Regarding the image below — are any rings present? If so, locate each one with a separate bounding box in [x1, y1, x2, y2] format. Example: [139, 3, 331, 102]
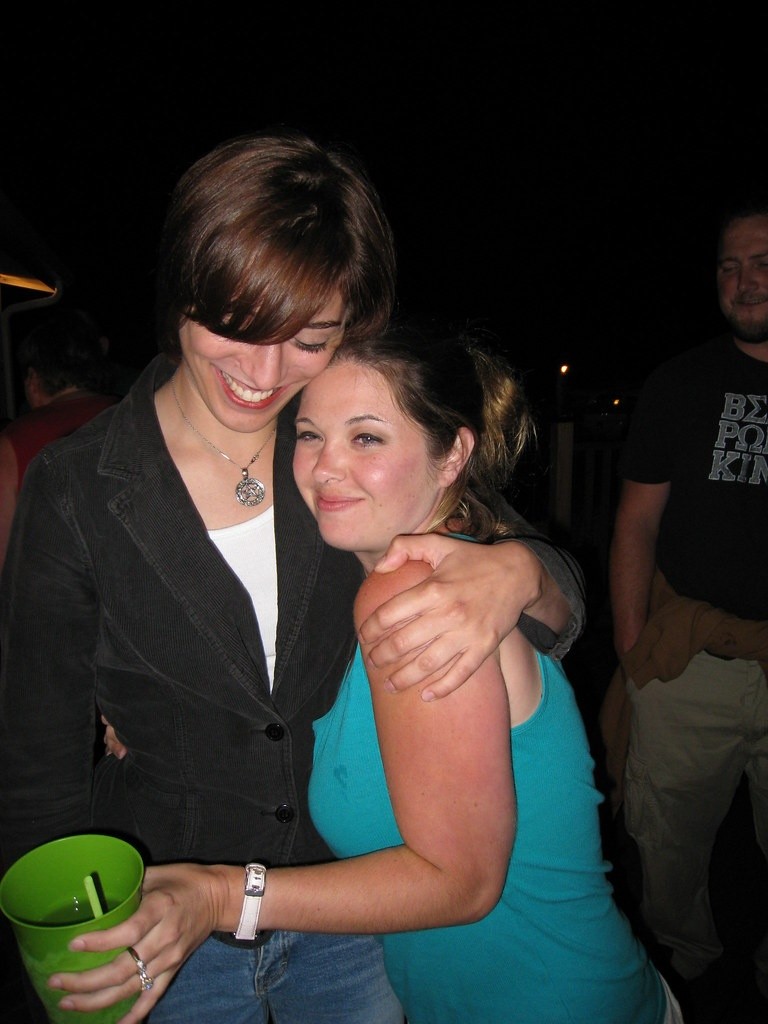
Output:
[126, 946, 145, 975]
[137, 969, 152, 990]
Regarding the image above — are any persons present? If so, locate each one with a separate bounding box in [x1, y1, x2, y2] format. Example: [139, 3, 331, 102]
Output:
[0, 310, 143, 592]
[604, 204, 768, 1024]
[0, 140, 583, 1024]
[48, 328, 683, 1024]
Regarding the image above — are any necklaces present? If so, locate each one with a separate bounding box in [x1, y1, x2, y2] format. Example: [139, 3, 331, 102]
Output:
[169, 375, 278, 507]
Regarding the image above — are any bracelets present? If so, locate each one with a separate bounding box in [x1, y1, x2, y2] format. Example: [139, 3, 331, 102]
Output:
[234, 862, 266, 941]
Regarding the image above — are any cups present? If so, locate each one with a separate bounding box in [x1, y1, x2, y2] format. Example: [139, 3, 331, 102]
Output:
[0, 836, 140, 1024]
